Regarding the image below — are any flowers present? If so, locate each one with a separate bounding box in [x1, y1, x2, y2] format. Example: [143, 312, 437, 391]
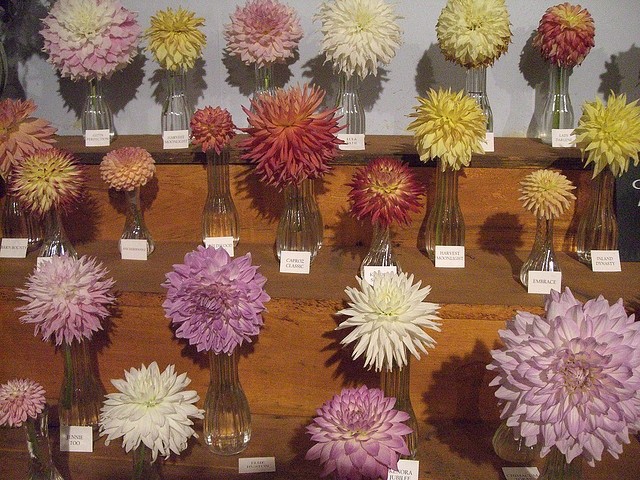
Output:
[14, 250, 124, 413]
[403, 85, 489, 245]
[333, 270, 443, 411]
[570, 88, 640, 253]
[487, 288, 640, 480]
[6, 148, 91, 256]
[435, 0, 513, 110]
[517, 170, 577, 269]
[0, 377, 46, 480]
[343, 154, 426, 252]
[38, 0, 143, 129]
[160, 245, 271, 435]
[189, 105, 236, 211]
[532, 2, 596, 129]
[304, 384, 414, 480]
[98, 361, 205, 480]
[0, 98, 59, 237]
[233, 81, 350, 249]
[312, 0, 405, 121]
[222, 0, 304, 91]
[100, 146, 156, 239]
[143, 5, 207, 116]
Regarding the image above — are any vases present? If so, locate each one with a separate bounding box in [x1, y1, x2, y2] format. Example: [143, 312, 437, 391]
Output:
[577, 169, 619, 264]
[426, 157, 465, 260]
[275, 179, 323, 266]
[330, 73, 365, 150]
[533, 446, 583, 480]
[541, 67, 574, 145]
[203, 349, 252, 455]
[57, 338, 108, 431]
[131, 443, 160, 480]
[118, 187, 154, 257]
[492, 417, 545, 464]
[2, 183, 45, 252]
[360, 222, 402, 278]
[81, 81, 115, 143]
[161, 72, 194, 145]
[462, 67, 493, 132]
[520, 216, 561, 288]
[37, 207, 78, 261]
[380, 350, 419, 459]
[249, 62, 275, 128]
[203, 149, 240, 247]
[22, 406, 65, 480]
